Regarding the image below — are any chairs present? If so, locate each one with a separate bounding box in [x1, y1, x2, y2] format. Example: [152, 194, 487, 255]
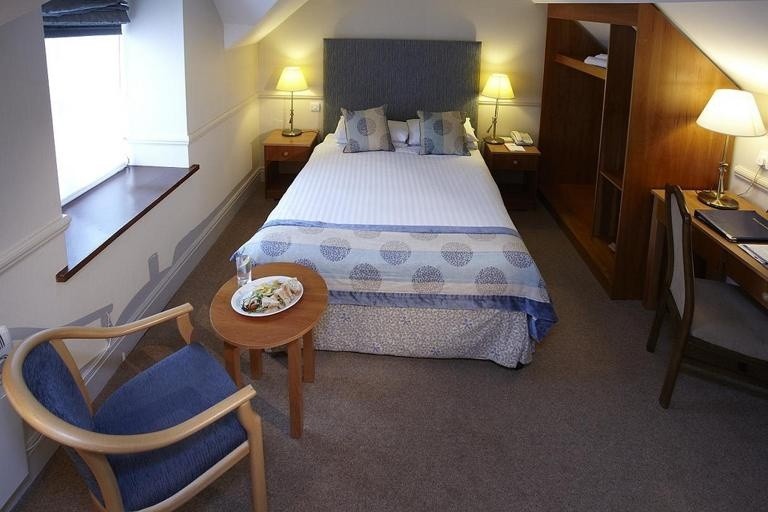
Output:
[2, 302, 268, 511]
[646, 183, 767, 410]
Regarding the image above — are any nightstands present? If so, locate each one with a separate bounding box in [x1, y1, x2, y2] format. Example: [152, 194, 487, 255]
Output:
[482, 137, 541, 212]
[261, 129, 317, 202]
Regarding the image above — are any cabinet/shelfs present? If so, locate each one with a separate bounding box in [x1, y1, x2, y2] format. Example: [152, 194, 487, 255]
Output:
[536, 5, 741, 302]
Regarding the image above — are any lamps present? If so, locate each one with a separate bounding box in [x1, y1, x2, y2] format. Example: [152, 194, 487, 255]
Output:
[481, 73, 516, 145]
[274, 66, 309, 137]
[694, 88, 767, 211]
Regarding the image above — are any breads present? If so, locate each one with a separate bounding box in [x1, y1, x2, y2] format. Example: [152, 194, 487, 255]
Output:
[257, 277, 300, 313]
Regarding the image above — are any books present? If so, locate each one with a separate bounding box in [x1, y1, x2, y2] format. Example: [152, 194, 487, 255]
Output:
[503, 142, 526, 153]
[736, 242, 768, 269]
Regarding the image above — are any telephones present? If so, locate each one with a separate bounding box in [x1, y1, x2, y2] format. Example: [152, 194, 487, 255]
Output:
[511, 131, 533, 146]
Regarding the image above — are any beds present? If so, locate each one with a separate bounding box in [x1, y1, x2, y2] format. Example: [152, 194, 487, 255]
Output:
[235, 118, 541, 372]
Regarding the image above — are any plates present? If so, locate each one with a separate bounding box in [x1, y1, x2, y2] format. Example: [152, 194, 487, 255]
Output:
[230, 275, 304, 318]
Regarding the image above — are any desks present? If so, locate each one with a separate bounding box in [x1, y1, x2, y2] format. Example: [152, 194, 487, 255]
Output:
[208, 262, 331, 441]
[641, 188, 767, 311]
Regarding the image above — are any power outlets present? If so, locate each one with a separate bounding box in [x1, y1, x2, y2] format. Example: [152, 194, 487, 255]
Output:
[754, 149, 767, 171]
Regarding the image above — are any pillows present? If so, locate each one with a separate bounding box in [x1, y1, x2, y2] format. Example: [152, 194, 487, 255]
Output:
[332, 103, 479, 157]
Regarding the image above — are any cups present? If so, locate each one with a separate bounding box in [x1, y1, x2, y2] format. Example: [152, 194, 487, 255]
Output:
[235, 254, 252, 288]
